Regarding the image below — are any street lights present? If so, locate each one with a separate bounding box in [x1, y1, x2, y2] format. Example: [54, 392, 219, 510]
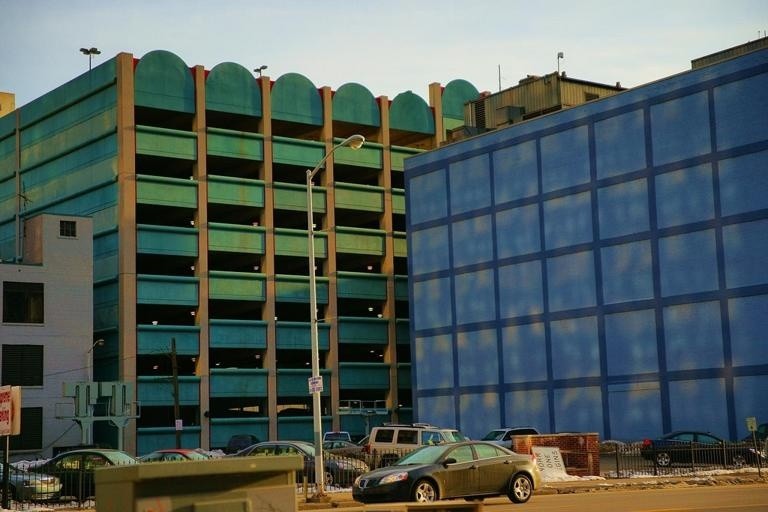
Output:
[306, 131, 367, 500]
[87, 337, 106, 444]
[79, 47, 101, 70]
[253, 65, 268, 77]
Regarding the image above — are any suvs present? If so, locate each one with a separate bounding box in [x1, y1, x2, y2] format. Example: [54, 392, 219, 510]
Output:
[364, 423, 468, 469]
[477, 425, 542, 448]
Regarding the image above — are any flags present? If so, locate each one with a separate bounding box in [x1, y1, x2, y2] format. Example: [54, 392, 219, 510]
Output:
[558, 53, 563, 58]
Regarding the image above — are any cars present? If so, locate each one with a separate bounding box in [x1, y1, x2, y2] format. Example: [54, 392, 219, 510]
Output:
[640, 428, 766, 469]
[27, 447, 141, 502]
[230, 438, 367, 488]
[321, 430, 353, 444]
[134, 447, 211, 462]
[0, 459, 65, 505]
[339, 398, 387, 410]
[351, 439, 542, 506]
